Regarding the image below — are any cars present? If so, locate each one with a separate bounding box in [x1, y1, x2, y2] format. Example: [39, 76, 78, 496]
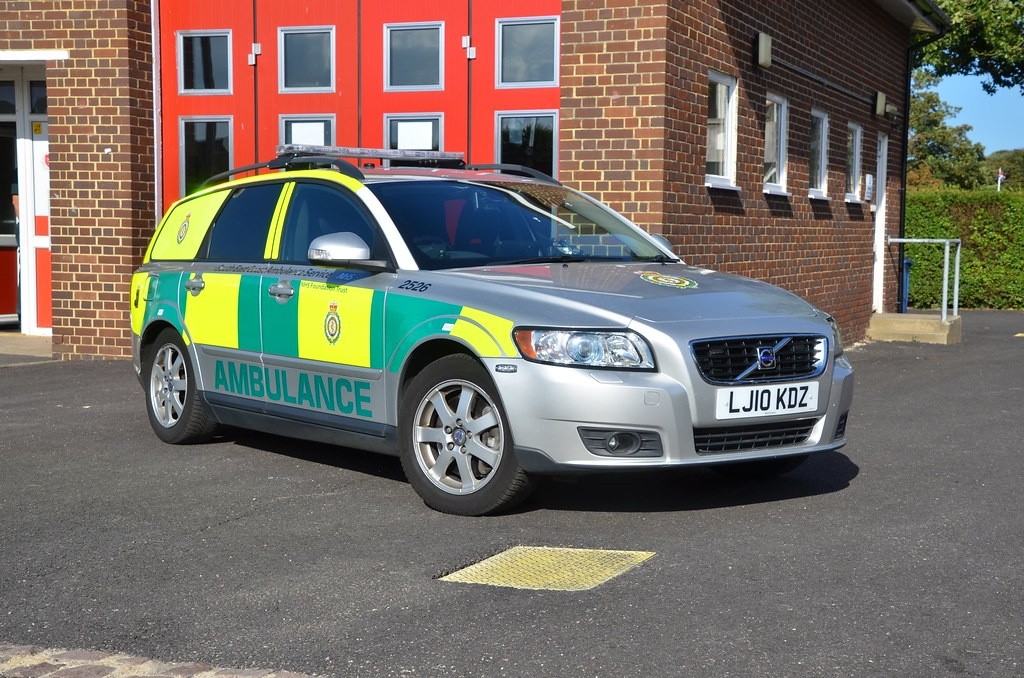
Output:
[129, 144, 856, 517]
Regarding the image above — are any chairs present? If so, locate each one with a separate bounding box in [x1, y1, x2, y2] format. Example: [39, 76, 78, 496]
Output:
[304, 195, 504, 267]
